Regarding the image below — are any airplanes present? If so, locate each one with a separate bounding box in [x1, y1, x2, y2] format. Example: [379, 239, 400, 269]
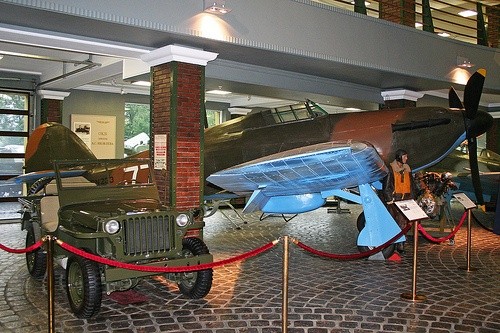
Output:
[22, 69, 494, 217]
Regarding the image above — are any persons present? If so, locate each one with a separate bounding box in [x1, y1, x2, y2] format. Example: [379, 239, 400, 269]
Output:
[385, 150, 416, 255]
[76, 125, 90, 132]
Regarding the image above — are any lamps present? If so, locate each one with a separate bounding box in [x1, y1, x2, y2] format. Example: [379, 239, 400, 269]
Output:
[456, 56, 476, 68]
[203, 1, 233, 15]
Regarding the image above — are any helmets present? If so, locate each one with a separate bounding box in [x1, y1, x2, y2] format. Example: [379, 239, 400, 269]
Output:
[395, 149, 407, 164]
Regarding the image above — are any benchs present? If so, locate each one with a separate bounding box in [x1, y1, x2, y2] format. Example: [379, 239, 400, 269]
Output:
[39, 176, 96, 231]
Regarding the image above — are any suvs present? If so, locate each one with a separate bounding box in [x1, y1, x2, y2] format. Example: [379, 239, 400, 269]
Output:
[17, 158, 213, 320]
